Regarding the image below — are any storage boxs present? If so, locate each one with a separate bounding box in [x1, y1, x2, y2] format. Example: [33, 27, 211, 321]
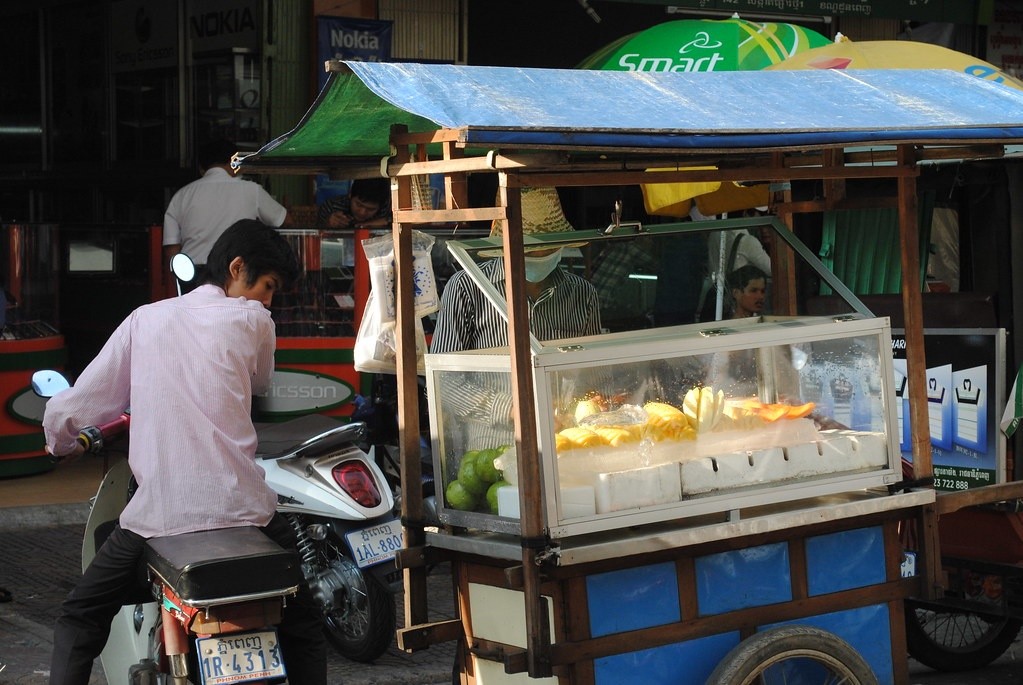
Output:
[595, 461, 680, 514]
[498, 485, 596, 522]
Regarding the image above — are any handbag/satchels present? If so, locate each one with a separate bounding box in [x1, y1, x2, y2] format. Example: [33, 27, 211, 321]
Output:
[354, 287, 428, 376]
[698, 232, 745, 323]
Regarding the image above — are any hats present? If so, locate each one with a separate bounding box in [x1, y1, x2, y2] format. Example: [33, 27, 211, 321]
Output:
[477, 185, 589, 257]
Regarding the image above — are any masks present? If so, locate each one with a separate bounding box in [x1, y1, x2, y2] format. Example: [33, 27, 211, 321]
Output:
[524, 246, 563, 283]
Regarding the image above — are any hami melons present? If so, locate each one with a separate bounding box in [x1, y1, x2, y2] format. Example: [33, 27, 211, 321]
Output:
[743, 399, 815, 423]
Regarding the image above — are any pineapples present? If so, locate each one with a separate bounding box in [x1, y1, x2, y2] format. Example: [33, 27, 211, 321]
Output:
[554, 397, 698, 452]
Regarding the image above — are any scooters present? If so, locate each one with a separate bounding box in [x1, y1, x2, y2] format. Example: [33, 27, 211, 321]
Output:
[253, 414, 409, 662]
[32, 254, 304, 685]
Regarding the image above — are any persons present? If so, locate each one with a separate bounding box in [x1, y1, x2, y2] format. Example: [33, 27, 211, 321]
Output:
[757, 225, 822, 316]
[316, 178, 395, 294]
[162, 141, 294, 297]
[694, 209, 772, 323]
[584, 236, 657, 321]
[423, 184, 617, 451]
[726, 266, 767, 319]
[41, 218, 330, 685]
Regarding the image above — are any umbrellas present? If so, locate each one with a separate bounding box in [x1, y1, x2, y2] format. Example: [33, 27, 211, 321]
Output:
[641, 31, 1023, 217]
[575, 20, 835, 71]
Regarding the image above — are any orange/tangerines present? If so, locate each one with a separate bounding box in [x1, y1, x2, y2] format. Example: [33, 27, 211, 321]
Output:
[446, 444, 509, 515]
